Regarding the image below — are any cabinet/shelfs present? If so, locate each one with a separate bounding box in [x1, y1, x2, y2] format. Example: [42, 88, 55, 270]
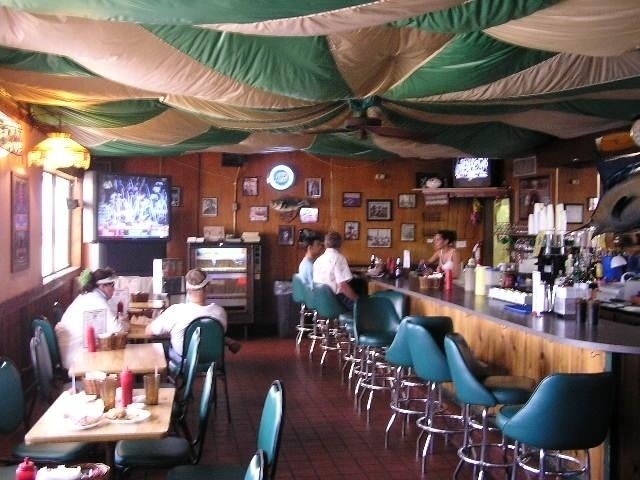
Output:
[368, 276, 640, 480]
[89, 242, 166, 276]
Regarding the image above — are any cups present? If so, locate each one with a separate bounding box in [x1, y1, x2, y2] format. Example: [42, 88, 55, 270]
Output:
[144, 373, 160, 404]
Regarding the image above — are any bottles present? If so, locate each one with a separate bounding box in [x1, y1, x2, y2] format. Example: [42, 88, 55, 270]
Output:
[464, 258, 475, 292]
[370, 254, 375, 269]
[395, 258, 401, 278]
[121, 366, 133, 406]
[15, 457, 35, 480]
[87, 321, 95, 352]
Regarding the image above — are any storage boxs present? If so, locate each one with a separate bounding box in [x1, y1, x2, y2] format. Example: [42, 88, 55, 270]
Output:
[513, 156, 544, 176]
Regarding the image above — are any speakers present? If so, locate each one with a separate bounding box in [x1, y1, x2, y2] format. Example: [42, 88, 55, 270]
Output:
[489, 156, 505, 187]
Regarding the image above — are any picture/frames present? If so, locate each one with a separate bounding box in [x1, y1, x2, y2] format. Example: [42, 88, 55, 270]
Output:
[10, 170, 30, 273]
[400, 223, 416, 242]
[304, 177, 321, 198]
[367, 199, 393, 221]
[344, 221, 360, 240]
[242, 176, 259, 196]
[564, 203, 584, 224]
[367, 228, 392, 248]
[299, 208, 318, 223]
[201, 198, 217, 217]
[398, 193, 417, 209]
[278, 225, 295, 245]
[518, 174, 551, 220]
[171, 187, 180, 207]
[248, 205, 269, 222]
[343, 192, 361, 207]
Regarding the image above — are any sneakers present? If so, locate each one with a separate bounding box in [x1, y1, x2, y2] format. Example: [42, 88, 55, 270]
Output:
[229, 342, 241, 354]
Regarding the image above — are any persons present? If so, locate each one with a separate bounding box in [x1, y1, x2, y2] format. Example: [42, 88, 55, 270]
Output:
[419, 229, 464, 280]
[146, 266, 242, 375]
[54, 266, 131, 383]
[313, 232, 360, 312]
[299, 236, 323, 291]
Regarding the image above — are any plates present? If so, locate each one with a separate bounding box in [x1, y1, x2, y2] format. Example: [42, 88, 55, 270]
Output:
[104, 409, 151, 423]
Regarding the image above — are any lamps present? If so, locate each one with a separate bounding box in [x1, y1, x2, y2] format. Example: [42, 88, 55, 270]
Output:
[266, 162, 296, 192]
[27, 113, 90, 169]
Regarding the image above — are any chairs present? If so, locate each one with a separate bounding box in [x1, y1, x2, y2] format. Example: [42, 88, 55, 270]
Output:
[172, 327, 201, 460]
[312, 282, 349, 369]
[168, 380, 285, 480]
[339, 312, 372, 390]
[292, 273, 314, 352]
[244, 450, 267, 480]
[30, 326, 71, 416]
[168, 317, 232, 425]
[303, 285, 325, 360]
[385, 316, 453, 449]
[115, 362, 216, 472]
[405, 321, 489, 478]
[496, 371, 615, 480]
[30, 316, 77, 389]
[354, 297, 411, 425]
[346, 290, 405, 409]
[0, 359, 99, 466]
[54, 302, 62, 322]
[445, 333, 536, 480]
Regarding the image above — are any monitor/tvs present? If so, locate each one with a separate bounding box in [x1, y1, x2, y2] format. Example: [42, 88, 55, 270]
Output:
[96, 171, 172, 244]
[451, 154, 492, 188]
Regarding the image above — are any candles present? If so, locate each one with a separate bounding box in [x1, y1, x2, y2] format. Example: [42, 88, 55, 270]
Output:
[446, 269, 453, 290]
[87, 324, 97, 352]
[119, 364, 133, 407]
[16, 458, 36, 479]
[117, 300, 123, 318]
